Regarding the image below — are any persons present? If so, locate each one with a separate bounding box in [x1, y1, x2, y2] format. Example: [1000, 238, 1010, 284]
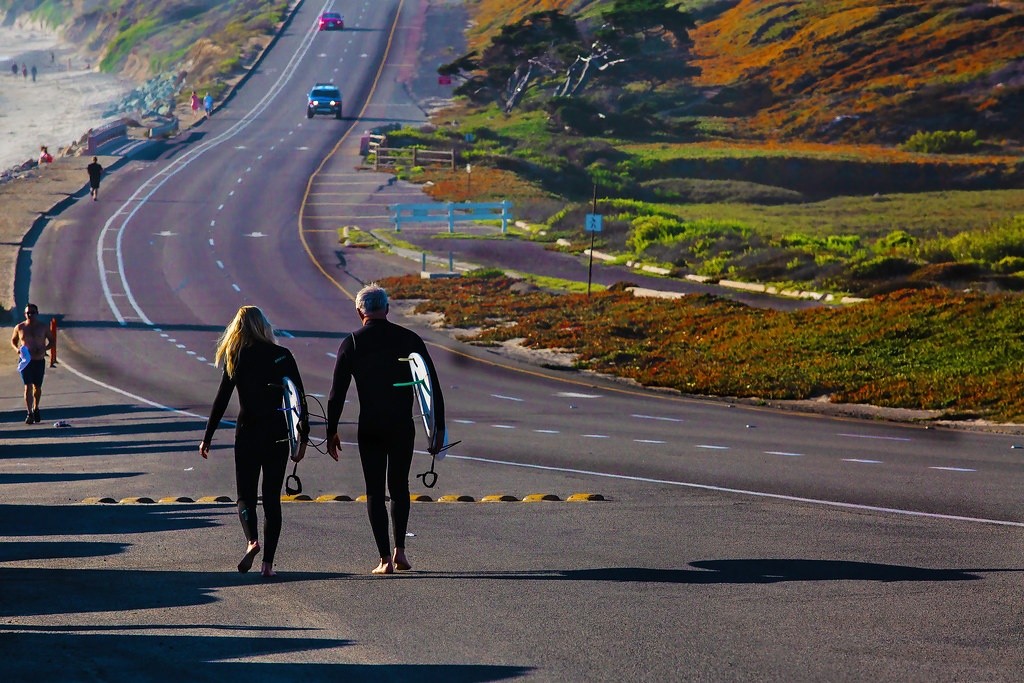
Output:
[325, 284, 447, 575]
[190, 91, 200, 119]
[11, 52, 91, 87]
[11, 302, 56, 425]
[87, 156, 104, 201]
[199, 306, 310, 579]
[37, 145, 53, 166]
[203, 91, 213, 120]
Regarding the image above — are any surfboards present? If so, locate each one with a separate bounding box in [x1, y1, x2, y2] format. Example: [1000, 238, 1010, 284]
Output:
[267, 377, 302, 459]
[392, 352, 450, 462]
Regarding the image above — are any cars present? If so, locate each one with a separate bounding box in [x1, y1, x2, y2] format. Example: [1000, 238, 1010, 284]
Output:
[317, 12, 344, 31]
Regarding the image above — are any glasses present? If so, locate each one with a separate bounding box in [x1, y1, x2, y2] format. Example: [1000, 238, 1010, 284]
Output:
[27, 312, 35, 314]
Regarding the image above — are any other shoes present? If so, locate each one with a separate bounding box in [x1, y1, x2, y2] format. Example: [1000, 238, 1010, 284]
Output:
[34, 410, 40, 422]
[25, 413, 34, 424]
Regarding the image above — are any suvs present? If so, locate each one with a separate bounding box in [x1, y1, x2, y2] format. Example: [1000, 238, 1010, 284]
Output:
[306, 84, 345, 118]
